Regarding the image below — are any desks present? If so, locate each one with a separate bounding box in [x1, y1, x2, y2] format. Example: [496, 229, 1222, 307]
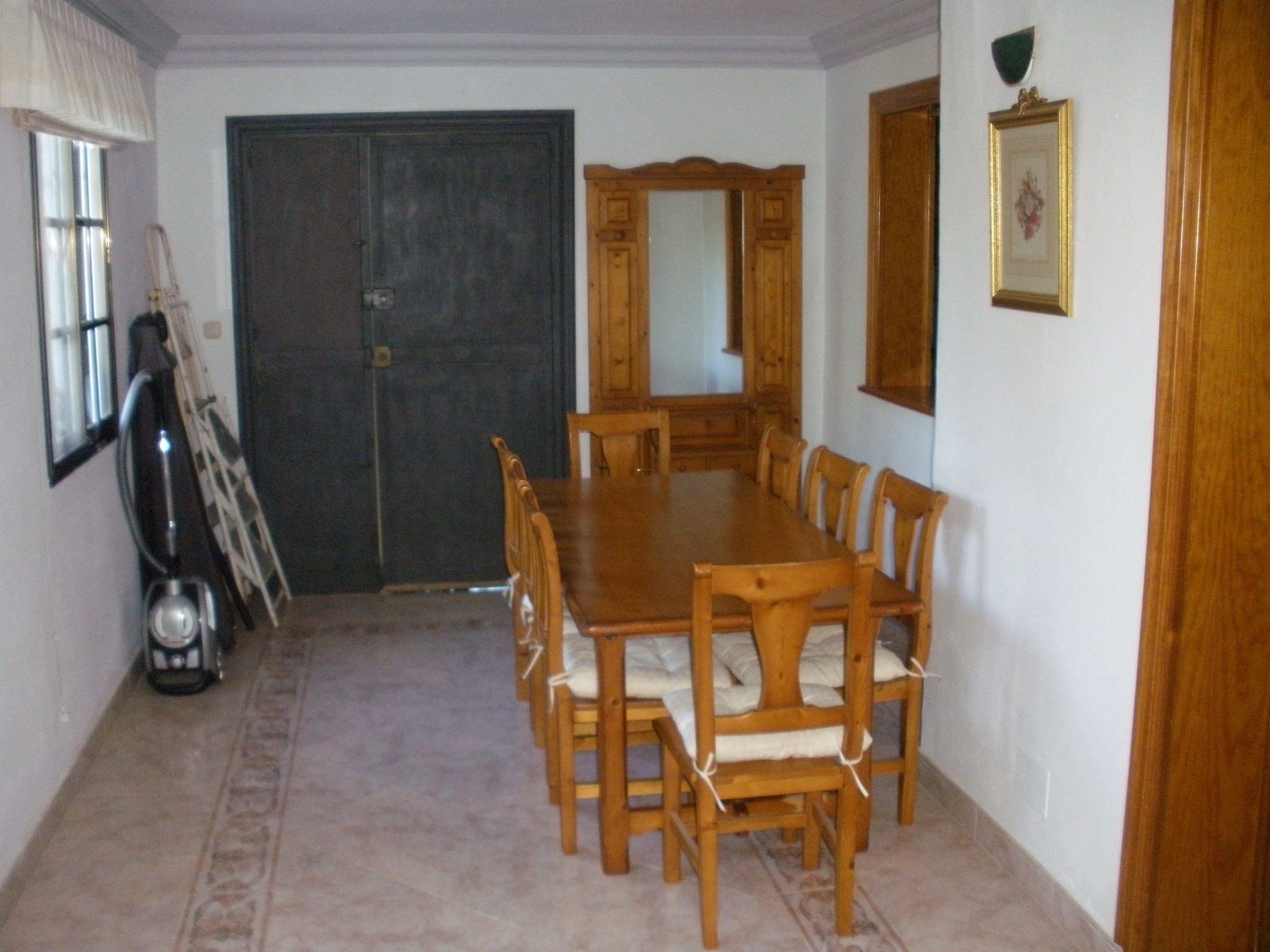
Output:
[522, 468, 927, 875]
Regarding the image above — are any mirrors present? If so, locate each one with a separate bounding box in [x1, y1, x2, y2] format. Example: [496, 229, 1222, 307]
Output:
[647, 187, 746, 399]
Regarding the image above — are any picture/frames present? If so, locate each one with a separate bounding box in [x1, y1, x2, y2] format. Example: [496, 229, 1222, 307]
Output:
[988, 85, 1074, 318]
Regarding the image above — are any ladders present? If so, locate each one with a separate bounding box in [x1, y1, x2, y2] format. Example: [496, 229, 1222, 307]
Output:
[144, 222, 292, 627]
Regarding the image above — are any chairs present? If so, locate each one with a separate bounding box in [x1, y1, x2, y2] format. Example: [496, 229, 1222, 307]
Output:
[489, 408, 949, 952]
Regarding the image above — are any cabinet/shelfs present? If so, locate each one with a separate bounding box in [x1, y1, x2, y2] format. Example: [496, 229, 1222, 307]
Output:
[587, 157, 806, 481]
